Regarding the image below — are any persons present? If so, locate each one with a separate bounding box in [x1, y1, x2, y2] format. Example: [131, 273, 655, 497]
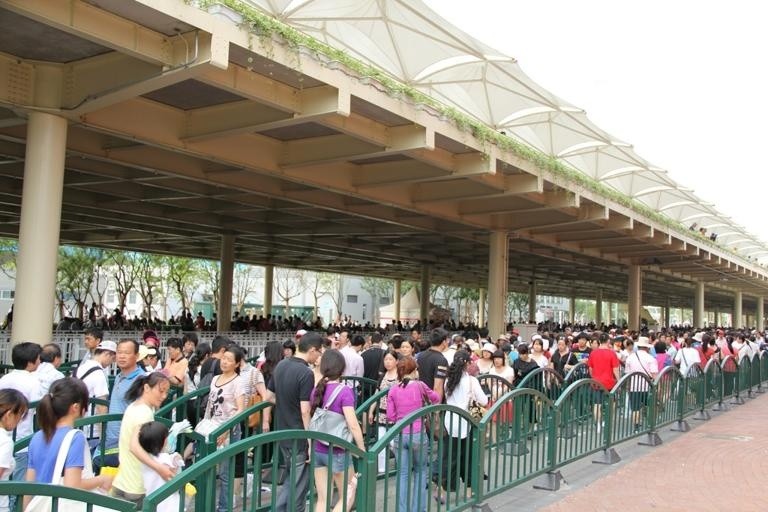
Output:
[689, 222, 763, 268]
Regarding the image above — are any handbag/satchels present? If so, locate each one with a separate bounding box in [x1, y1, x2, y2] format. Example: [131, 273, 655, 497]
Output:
[466, 371, 488, 424]
[193, 418, 226, 451]
[197, 358, 220, 412]
[306, 383, 354, 449]
[563, 363, 580, 380]
[415, 380, 450, 440]
[21, 428, 122, 512]
[245, 392, 261, 427]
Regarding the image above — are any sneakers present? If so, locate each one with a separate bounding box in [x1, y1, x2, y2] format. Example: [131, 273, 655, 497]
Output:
[465, 496, 475, 502]
[633, 424, 640, 433]
[596, 421, 605, 434]
[433, 490, 445, 505]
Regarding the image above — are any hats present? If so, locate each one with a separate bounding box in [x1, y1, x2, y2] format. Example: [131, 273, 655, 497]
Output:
[636, 336, 654, 349]
[96, 340, 118, 353]
[496, 327, 521, 343]
[691, 332, 703, 343]
[136, 344, 157, 362]
[480, 342, 498, 354]
[295, 328, 308, 340]
[529, 334, 550, 350]
